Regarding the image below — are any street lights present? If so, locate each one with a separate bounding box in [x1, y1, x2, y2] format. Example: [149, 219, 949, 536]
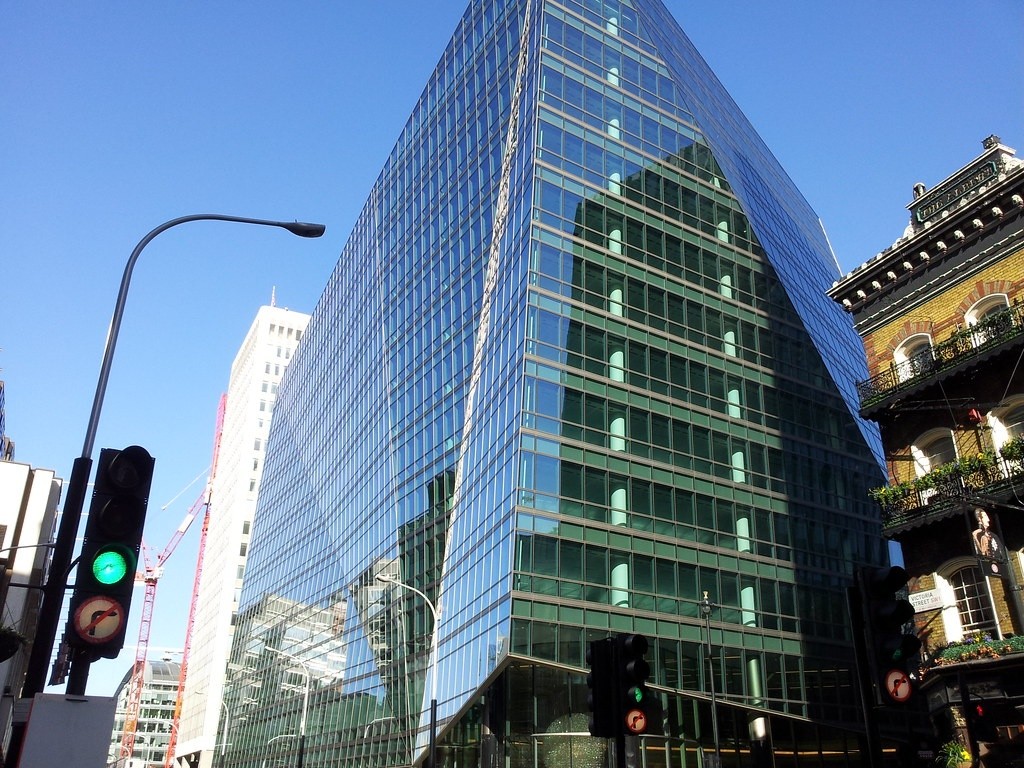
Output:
[110, 718, 180, 768]
[195, 689, 230, 768]
[265, 646, 309, 765]
[375, 572, 439, 768]
[0, 212, 326, 768]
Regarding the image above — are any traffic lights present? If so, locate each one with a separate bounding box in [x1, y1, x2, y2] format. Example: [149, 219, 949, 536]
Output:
[868, 564, 924, 683]
[611, 633, 650, 739]
[70, 446, 159, 664]
